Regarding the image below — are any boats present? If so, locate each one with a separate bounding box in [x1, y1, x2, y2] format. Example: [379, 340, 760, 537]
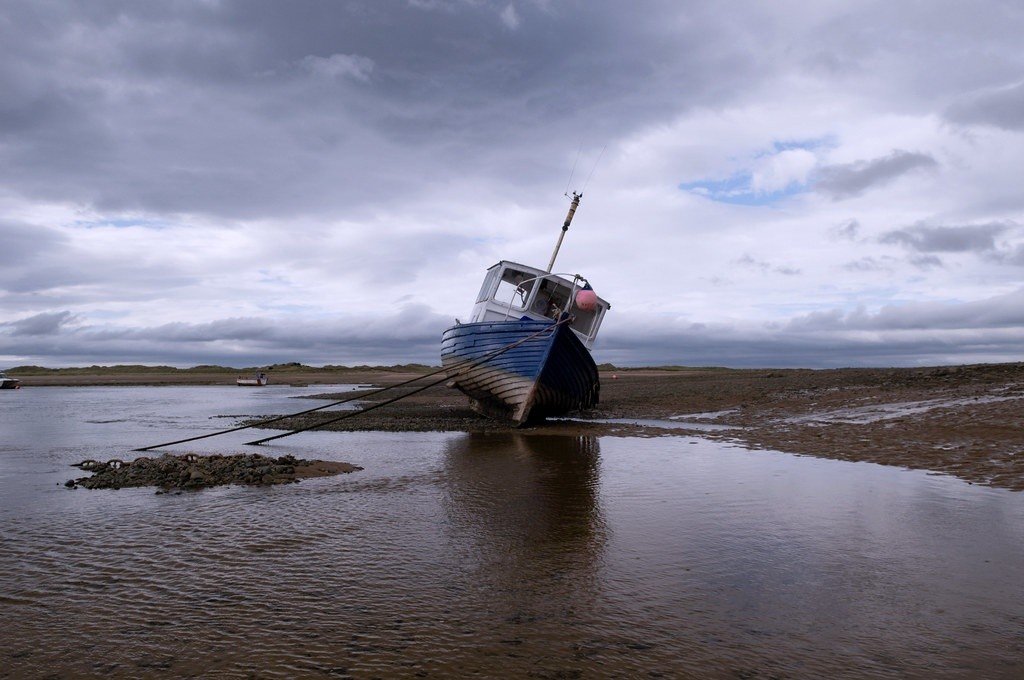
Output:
[0, 373, 21, 390]
[440, 179, 616, 430]
[236, 376, 268, 386]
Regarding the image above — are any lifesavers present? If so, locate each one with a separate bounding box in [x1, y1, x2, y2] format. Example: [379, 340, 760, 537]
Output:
[534, 298, 546, 311]
